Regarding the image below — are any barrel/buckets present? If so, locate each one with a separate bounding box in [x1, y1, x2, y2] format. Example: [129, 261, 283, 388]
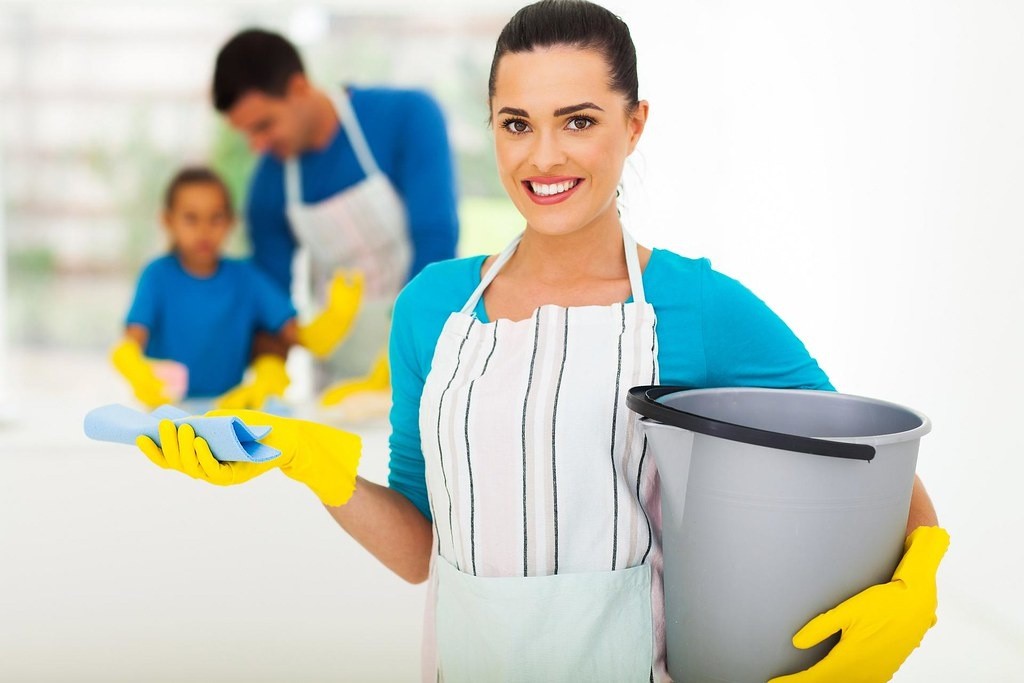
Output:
[625, 384, 933, 683]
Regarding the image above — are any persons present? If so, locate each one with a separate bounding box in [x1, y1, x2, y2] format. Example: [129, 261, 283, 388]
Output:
[136, 1, 950, 683]
[109, 170, 362, 410]
[209, 28, 460, 410]
